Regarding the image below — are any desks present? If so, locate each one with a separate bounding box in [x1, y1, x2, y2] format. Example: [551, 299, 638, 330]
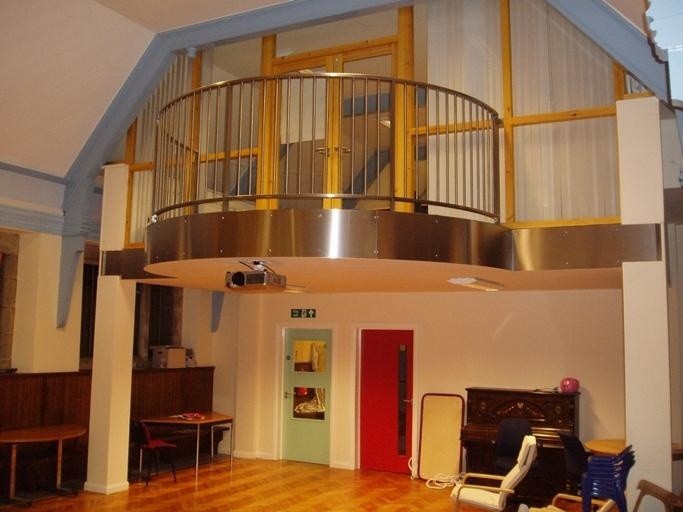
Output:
[582, 439, 683, 463]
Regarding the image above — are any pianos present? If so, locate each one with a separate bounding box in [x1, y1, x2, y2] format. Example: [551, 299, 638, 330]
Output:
[459, 387, 582, 507]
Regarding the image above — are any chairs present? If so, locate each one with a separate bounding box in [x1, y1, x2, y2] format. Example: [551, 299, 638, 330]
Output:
[491, 416, 531, 505]
[130, 420, 178, 487]
[450, 435, 538, 511]
[581, 445, 635, 512]
[557, 429, 591, 493]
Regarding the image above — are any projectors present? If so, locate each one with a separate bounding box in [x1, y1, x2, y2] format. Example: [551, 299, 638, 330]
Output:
[224, 270, 285, 293]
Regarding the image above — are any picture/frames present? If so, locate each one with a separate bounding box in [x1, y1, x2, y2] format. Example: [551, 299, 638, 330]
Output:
[292, 340, 326, 372]
[291, 386, 326, 422]
